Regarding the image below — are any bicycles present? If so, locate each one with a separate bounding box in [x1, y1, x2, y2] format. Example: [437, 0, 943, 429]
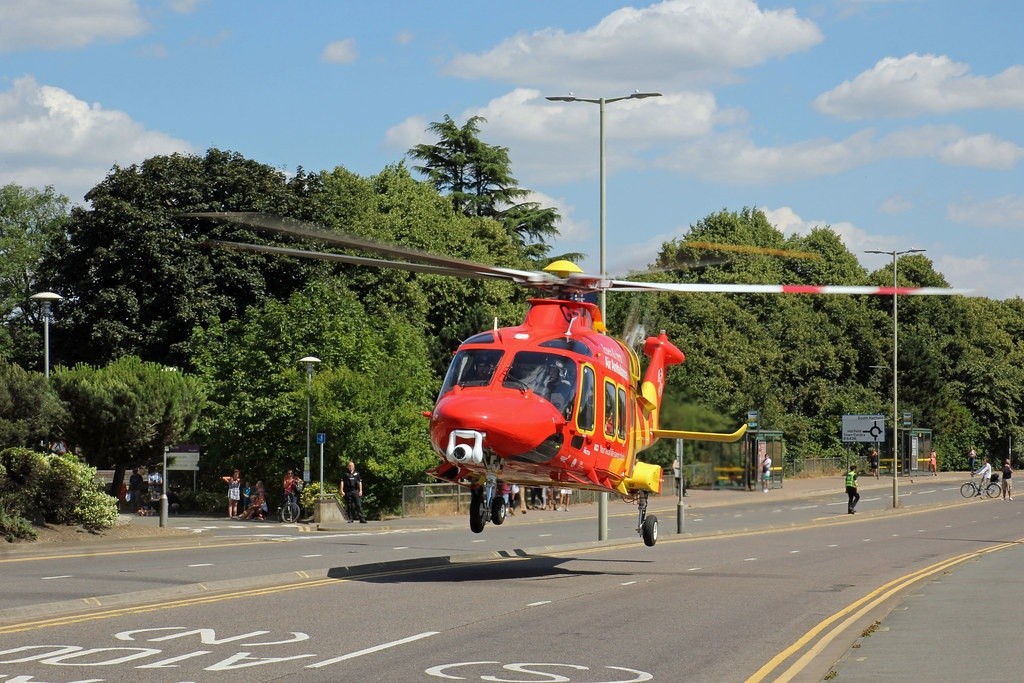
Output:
[960, 472, 1002, 498]
[280, 488, 301, 523]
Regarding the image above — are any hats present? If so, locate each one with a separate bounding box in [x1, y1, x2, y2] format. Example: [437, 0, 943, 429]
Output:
[850, 466, 856, 469]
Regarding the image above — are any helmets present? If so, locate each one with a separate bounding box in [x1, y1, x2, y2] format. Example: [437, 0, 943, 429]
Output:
[474, 355, 493, 375]
[548, 360, 567, 379]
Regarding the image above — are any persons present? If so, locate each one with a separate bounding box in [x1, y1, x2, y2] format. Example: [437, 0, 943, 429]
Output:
[632, 489, 638, 504]
[118, 483, 127, 510]
[237, 481, 268, 520]
[222, 469, 241, 519]
[340, 462, 367, 523]
[1001, 459, 1014, 500]
[929, 448, 937, 476]
[496, 483, 572, 515]
[845, 466, 860, 514]
[869, 448, 877, 477]
[968, 448, 975, 472]
[128, 469, 144, 513]
[974, 458, 991, 499]
[141, 488, 181, 515]
[284, 469, 303, 518]
[762, 454, 771, 493]
[673, 456, 687, 497]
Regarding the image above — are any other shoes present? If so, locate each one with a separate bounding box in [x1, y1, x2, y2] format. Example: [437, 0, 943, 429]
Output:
[849, 507, 856, 512]
[974, 493, 981, 497]
[935, 473, 937, 476]
[226, 513, 265, 522]
[683, 492, 689, 497]
[763, 488, 768, 493]
[505, 505, 570, 514]
[1009, 497, 1013, 501]
[348, 518, 353, 523]
[1001, 498, 1005, 500]
[360, 518, 367, 523]
[848, 509, 854, 514]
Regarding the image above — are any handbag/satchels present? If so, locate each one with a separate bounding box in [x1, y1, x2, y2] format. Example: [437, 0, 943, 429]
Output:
[990, 474, 999, 482]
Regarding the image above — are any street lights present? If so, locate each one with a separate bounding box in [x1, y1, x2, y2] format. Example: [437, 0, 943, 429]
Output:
[299, 356, 322, 519]
[863, 250, 929, 508]
[29, 291, 64, 382]
[544, 92, 666, 528]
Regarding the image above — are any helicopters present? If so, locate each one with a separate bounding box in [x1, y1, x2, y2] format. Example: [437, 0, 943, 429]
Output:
[177, 209, 1024, 547]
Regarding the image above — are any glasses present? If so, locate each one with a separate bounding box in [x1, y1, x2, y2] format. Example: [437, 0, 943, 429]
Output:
[287, 472, 293, 474]
